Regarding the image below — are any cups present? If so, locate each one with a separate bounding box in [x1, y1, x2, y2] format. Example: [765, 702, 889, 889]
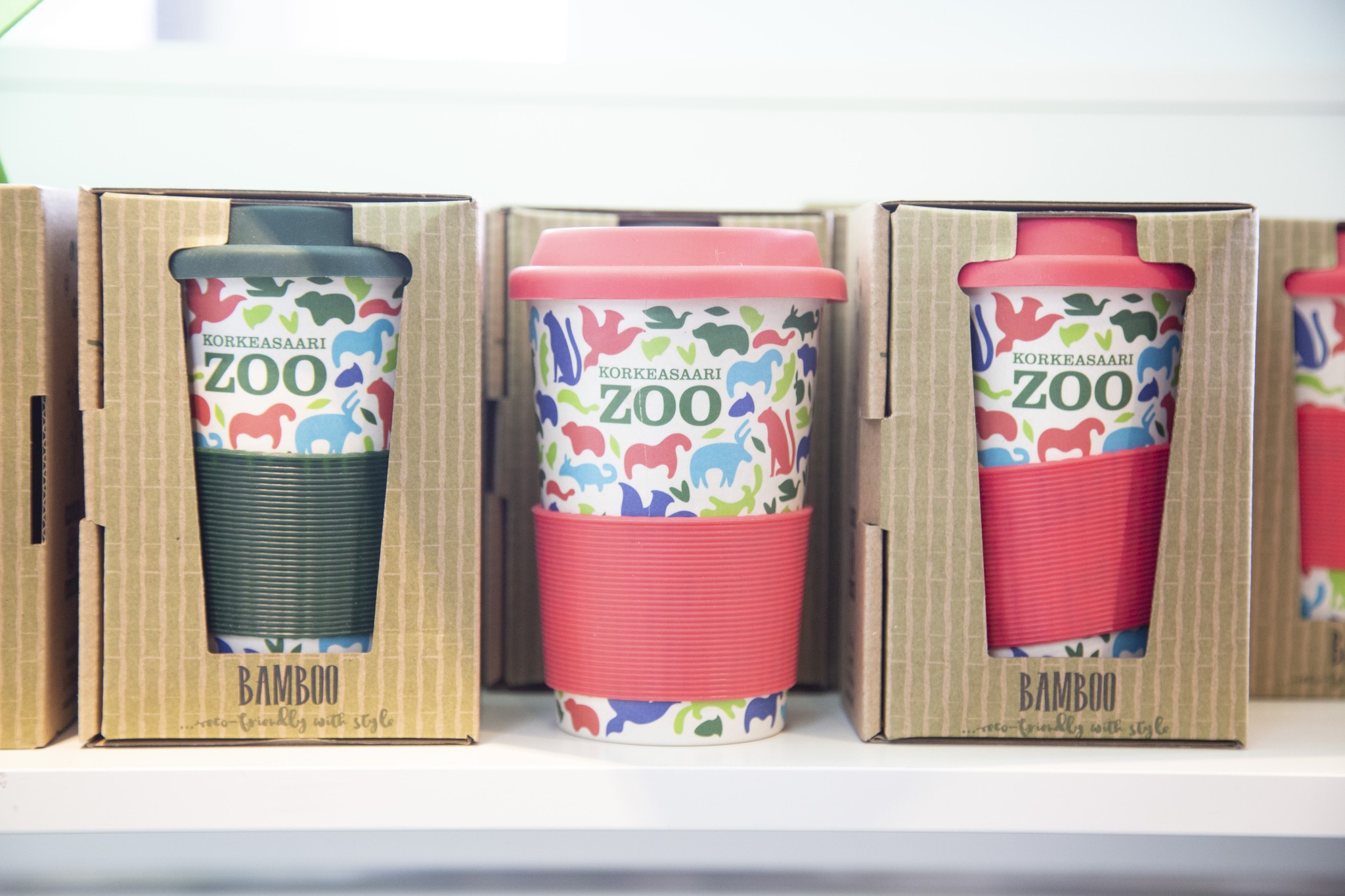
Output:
[504, 224, 848, 749]
[1284, 227, 1345, 628]
[168, 202, 412, 656]
[948, 216, 1195, 661]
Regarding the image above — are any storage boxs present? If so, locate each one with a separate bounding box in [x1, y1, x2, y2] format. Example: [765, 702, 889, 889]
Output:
[0, 181, 1345, 751]
